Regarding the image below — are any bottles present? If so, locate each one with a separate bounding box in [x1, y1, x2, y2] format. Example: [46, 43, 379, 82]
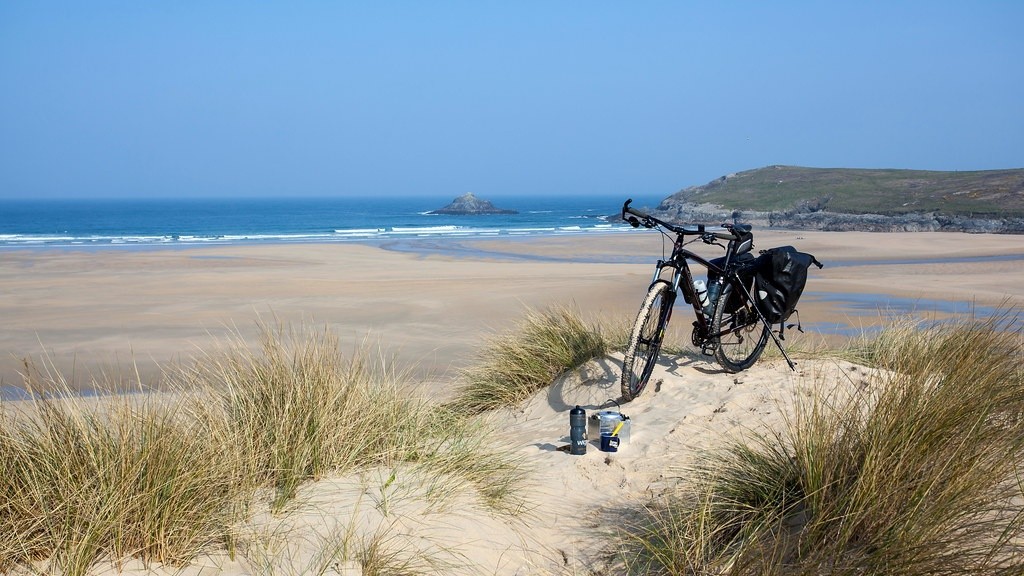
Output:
[569, 405, 587, 455]
[693, 280, 710, 307]
[704, 278, 721, 321]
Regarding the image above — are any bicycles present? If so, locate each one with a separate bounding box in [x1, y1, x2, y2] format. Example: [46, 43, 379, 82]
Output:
[621, 198, 774, 404]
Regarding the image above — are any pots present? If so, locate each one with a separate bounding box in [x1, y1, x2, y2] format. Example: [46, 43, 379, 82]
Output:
[588, 410, 625, 442]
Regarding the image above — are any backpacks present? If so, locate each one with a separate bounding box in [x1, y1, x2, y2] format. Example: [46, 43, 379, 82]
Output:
[754, 246, 823, 340]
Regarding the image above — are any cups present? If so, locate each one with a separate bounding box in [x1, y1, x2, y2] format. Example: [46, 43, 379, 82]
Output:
[618, 419, 630, 445]
[600, 431, 620, 452]
